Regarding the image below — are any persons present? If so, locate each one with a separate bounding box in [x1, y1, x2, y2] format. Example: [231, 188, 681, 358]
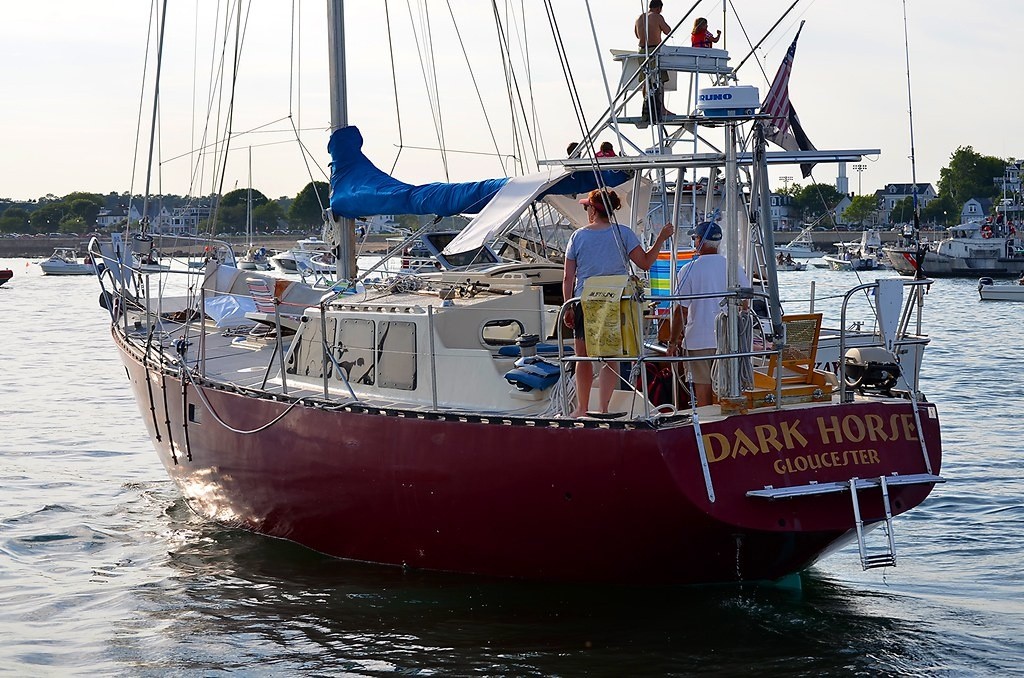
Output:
[986, 214, 994, 223]
[595, 141, 622, 158]
[249, 244, 256, 256]
[567, 143, 582, 157]
[785, 253, 791, 265]
[953, 230, 967, 238]
[996, 213, 1005, 225]
[857, 248, 861, 259]
[692, 17, 721, 49]
[634, 0, 676, 116]
[667, 221, 751, 407]
[561, 189, 673, 419]
[260, 245, 266, 256]
[777, 252, 783, 265]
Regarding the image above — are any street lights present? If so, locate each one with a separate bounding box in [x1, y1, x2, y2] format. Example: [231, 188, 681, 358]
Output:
[778, 176, 794, 193]
[852, 164, 868, 196]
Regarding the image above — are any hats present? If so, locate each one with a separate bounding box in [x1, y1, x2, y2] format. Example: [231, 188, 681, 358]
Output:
[687, 222, 722, 241]
[579, 196, 607, 214]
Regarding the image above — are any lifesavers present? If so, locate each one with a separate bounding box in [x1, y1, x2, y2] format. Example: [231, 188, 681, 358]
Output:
[981, 226, 991, 239]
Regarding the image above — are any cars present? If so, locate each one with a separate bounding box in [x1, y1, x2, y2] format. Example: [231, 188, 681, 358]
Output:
[873, 224, 882, 230]
[837, 224, 849, 231]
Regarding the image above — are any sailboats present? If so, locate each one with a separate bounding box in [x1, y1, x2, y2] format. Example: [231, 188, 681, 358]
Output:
[86, 0, 939, 585]
[236, 146, 270, 270]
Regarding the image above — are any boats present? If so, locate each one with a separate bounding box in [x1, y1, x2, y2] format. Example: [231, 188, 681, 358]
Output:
[882, 165, 1024, 278]
[977, 277, 1024, 302]
[189, 258, 237, 269]
[776, 261, 809, 272]
[128, 248, 171, 273]
[774, 224, 826, 258]
[1, 269, 14, 285]
[272, 237, 338, 274]
[384, 236, 443, 276]
[39, 249, 103, 275]
[824, 242, 878, 271]
[310, 253, 339, 275]
[811, 263, 829, 268]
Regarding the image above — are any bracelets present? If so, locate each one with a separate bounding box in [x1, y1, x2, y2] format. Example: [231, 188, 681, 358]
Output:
[562, 305, 572, 310]
[667, 340, 677, 347]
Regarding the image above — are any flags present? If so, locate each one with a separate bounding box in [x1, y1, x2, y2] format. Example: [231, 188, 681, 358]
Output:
[752, 35, 818, 178]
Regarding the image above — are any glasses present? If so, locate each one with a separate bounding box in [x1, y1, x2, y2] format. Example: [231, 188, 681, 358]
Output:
[582, 204, 589, 211]
[691, 236, 698, 240]
[704, 23, 708, 27]
